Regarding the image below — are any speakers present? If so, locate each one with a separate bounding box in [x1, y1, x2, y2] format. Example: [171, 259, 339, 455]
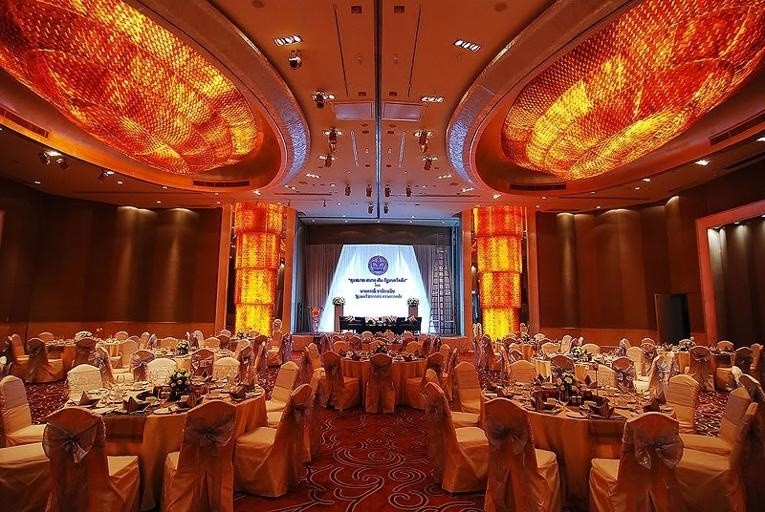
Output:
[654, 291, 691, 352]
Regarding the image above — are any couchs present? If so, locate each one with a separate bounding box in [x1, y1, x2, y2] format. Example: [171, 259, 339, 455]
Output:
[339, 314, 423, 335]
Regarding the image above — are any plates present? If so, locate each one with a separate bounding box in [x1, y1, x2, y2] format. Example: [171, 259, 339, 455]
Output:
[500, 377, 669, 419]
[63, 368, 258, 414]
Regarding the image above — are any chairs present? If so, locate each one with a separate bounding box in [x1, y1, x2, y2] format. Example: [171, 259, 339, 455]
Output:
[676, 387, 752, 455]
[158, 399, 240, 512]
[586, 412, 683, 512]
[234, 384, 313, 499]
[40, 405, 141, 512]
[0, 442, 50, 512]
[266, 368, 327, 464]
[482, 397, 561, 512]
[674, 402, 759, 511]
[0, 375, 46, 446]
[421, 382, 490, 493]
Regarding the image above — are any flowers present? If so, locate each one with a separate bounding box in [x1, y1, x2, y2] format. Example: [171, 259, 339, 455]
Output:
[332, 296, 345, 305]
[407, 298, 419, 307]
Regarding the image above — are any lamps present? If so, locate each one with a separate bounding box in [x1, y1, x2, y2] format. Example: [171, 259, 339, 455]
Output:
[471, 204, 526, 343]
[287, 48, 303, 70]
[498, 0, 764, 183]
[326, 125, 433, 215]
[312, 88, 326, 108]
[0, 0, 258, 177]
[232, 201, 284, 337]
